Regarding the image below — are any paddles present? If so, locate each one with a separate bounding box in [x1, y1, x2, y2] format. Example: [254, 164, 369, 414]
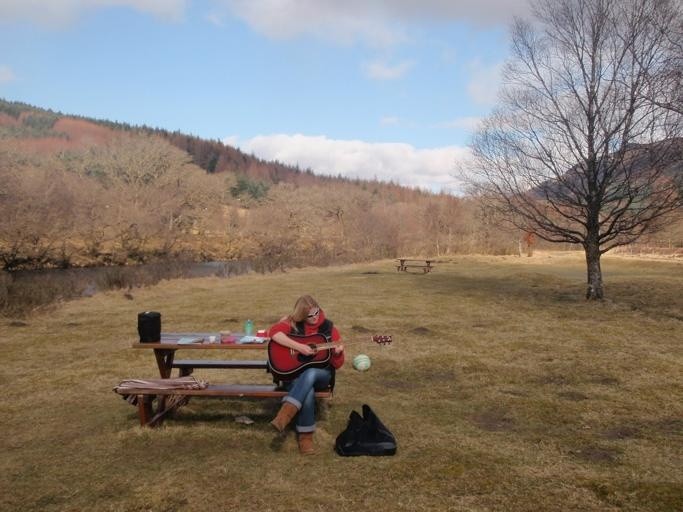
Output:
[267, 334, 392, 375]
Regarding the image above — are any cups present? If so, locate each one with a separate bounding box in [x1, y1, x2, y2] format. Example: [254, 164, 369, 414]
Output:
[209, 336, 216, 344]
[244, 321, 254, 337]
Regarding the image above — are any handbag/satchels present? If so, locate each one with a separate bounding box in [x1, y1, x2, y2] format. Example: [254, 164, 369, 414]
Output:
[334, 403, 398, 456]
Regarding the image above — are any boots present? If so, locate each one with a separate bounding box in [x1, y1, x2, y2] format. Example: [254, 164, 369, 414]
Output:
[298, 432, 316, 456]
[270, 401, 298, 433]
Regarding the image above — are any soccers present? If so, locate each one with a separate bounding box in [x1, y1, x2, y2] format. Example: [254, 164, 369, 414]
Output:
[353, 354, 370, 371]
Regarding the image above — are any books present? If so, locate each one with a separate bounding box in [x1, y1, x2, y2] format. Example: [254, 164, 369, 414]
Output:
[240, 335, 265, 344]
[177, 337, 204, 345]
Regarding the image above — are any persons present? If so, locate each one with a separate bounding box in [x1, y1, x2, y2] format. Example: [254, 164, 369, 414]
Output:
[265, 294, 345, 454]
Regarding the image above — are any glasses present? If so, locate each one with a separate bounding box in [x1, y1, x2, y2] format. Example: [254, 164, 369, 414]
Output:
[306, 311, 319, 318]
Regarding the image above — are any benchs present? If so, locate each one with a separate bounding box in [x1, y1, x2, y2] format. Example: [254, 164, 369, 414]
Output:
[395, 258, 433, 273]
[116, 331, 333, 429]
